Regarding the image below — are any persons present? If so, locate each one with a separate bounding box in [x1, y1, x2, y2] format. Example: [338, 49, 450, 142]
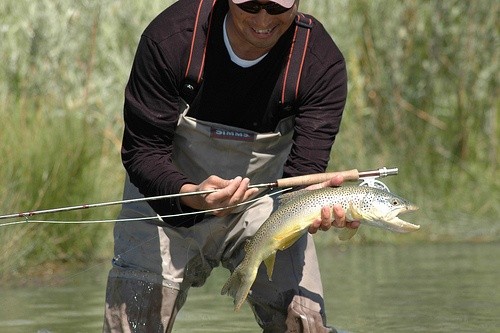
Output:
[103, 0, 361, 333]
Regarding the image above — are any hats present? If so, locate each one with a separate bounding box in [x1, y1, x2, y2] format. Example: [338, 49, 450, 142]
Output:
[231, 0, 295, 9]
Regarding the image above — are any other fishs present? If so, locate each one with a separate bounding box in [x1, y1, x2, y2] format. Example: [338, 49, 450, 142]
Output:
[220, 185, 421, 311]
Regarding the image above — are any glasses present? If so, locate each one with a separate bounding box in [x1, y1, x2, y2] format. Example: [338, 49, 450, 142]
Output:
[235, 0, 295, 15]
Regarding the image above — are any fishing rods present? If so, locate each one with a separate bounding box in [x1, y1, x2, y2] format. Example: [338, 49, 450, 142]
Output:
[1, 167, 399, 221]
